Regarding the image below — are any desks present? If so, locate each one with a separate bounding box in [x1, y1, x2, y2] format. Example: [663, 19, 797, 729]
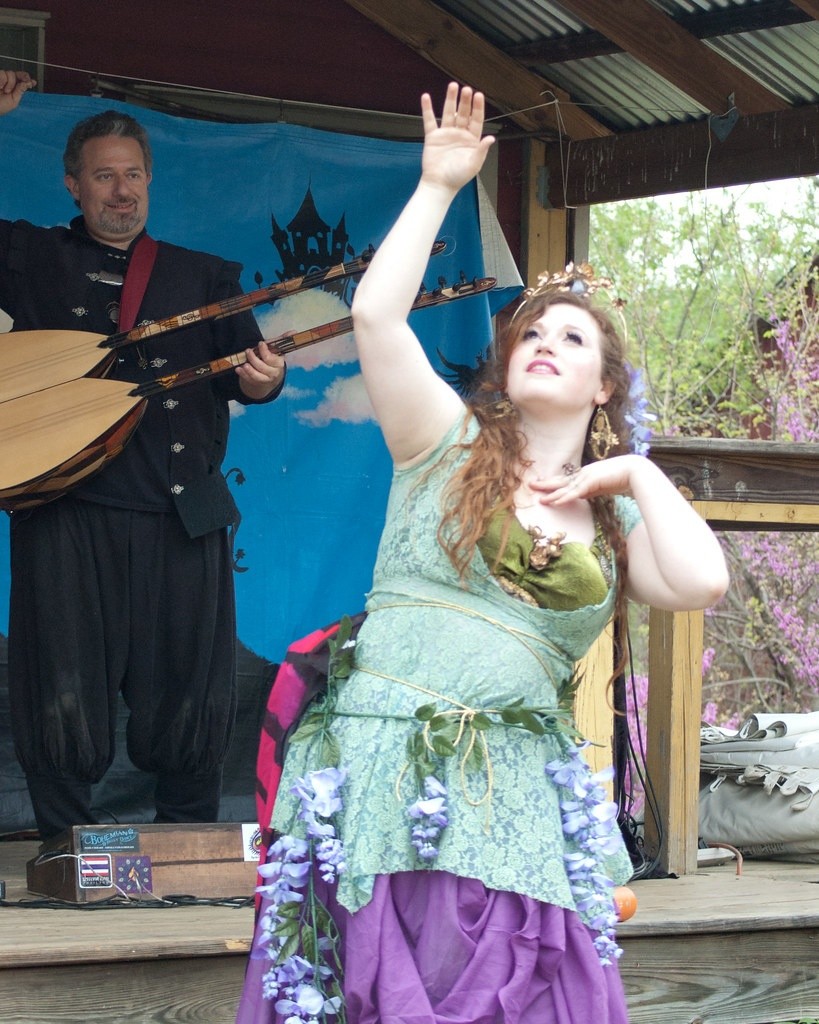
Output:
[628, 437, 819, 876]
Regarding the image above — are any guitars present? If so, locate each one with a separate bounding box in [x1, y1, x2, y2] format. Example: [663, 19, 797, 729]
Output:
[0, 238, 500, 514]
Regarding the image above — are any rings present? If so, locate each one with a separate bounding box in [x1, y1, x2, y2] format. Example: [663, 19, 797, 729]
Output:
[574, 480, 577, 487]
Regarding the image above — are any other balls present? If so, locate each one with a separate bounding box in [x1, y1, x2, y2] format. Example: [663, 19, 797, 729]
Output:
[613, 884, 638, 922]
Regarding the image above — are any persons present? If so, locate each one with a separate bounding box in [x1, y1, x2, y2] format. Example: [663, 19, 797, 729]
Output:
[0, 68, 287, 847]
[241, 81, 730, 1024]
